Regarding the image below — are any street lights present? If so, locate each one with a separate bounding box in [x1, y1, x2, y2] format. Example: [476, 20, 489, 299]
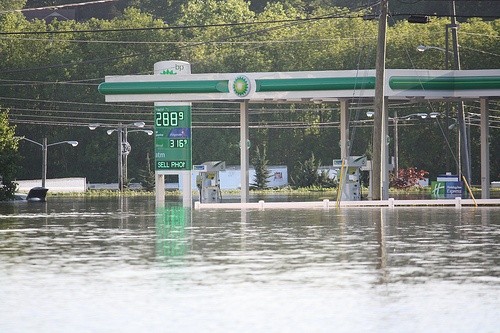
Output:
[366, 111, 428, 178]
[107, 129, 153, 182]
[13, 136, 78, 187]
[88, 122, 145, 192]
[430, 111, 462, 181]
